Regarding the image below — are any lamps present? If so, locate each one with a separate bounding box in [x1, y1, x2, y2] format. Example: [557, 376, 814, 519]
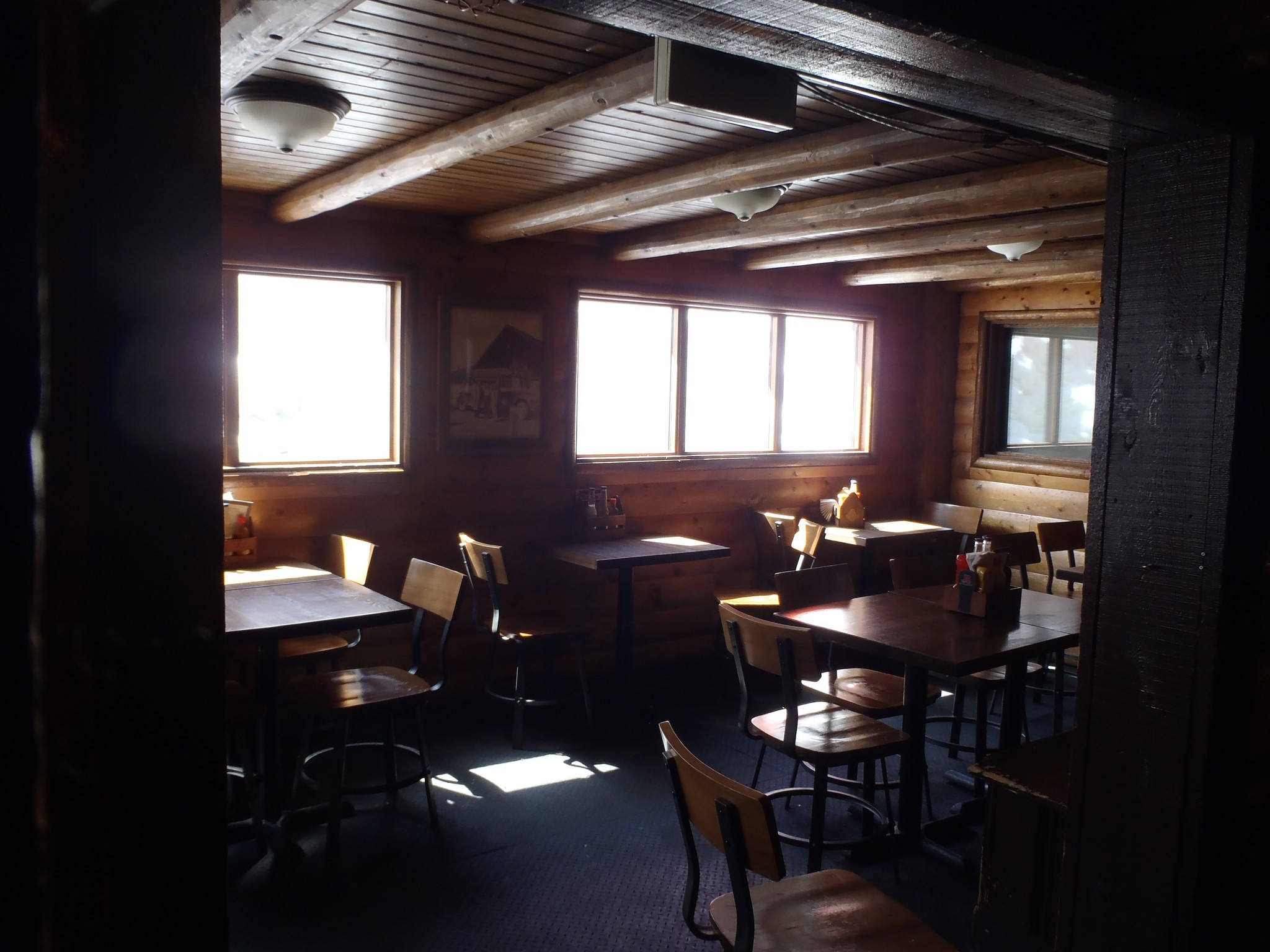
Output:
[711, 182, 790, 222]
[221, 81, 352, 152]
[986, 237, 1044, 262]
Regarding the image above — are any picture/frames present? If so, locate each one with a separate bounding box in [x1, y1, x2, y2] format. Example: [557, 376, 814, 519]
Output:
[435, 290, 555, 453]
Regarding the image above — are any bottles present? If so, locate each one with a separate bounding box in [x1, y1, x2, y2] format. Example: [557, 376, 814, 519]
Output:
[223, 503, 233, 556]
[233, 517, 250, 555]
[976, 535, 992, 592]
[585, 488, 596, 531]
[609, 498, 618, 528]
[850, 480, 860, 498]
[597, 486, 609, 530]
[973, 538, 983, 553]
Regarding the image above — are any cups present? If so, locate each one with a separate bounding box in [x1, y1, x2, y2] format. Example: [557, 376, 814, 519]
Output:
[955, 554, 970, 586]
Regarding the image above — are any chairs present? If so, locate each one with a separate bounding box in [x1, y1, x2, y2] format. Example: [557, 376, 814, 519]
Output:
[967, 734, 1075, 952]
[983, 520, 1087, 737]
[719, 602, 900, 883]
[655, 721, 957, 952]
[887, 502, 984, 590]
[708, 509, 828, 719]
[776, 561, 942, 845]
[277, 535, 465, 897]
[458, 529, 600, 750]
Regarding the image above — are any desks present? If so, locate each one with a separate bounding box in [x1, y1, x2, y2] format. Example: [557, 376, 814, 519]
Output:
[771, 581, 1084, 885]
[809, 517, 954, 597]
[224, 560, 411, 925]
[525, 532, 732, 759]
